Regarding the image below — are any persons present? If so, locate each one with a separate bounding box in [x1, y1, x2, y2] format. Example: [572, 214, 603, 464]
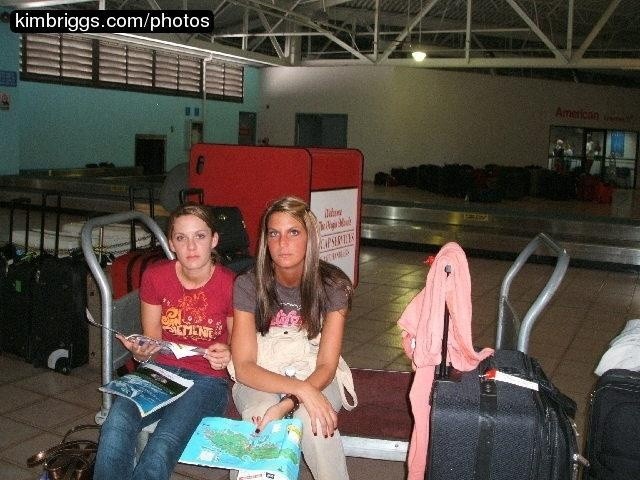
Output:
[553, 140, 564, 169]
[230, 195, 355, 479]
[594, 142, 601, 156]
[585, 134, 595, 158]
[92, 204, 236, 479]
[564, 141, 574, 170]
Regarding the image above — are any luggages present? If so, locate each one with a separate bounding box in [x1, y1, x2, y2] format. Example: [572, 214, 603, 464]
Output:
[0, 190, 115, 375]
[424, 264, 585, 480]
[582, 369, 639, 479]
[109, 182, 166, 373]
[180, 186, 259, 274]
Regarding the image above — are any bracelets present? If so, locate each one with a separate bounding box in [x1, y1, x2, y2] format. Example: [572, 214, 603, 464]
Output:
[132, 354, 153, 365]
[279, 392, 300, 419]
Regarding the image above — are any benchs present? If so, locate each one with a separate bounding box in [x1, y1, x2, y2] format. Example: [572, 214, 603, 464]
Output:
[79, 210, 569, 480]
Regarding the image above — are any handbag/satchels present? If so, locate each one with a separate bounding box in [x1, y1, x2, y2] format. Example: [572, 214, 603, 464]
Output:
[226, 326, 318, 386]
[41, 440, 97, 480]
[204, 206, 249, 256]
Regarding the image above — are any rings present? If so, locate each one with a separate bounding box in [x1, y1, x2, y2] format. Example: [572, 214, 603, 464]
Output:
[220, 363, 224, 370]
